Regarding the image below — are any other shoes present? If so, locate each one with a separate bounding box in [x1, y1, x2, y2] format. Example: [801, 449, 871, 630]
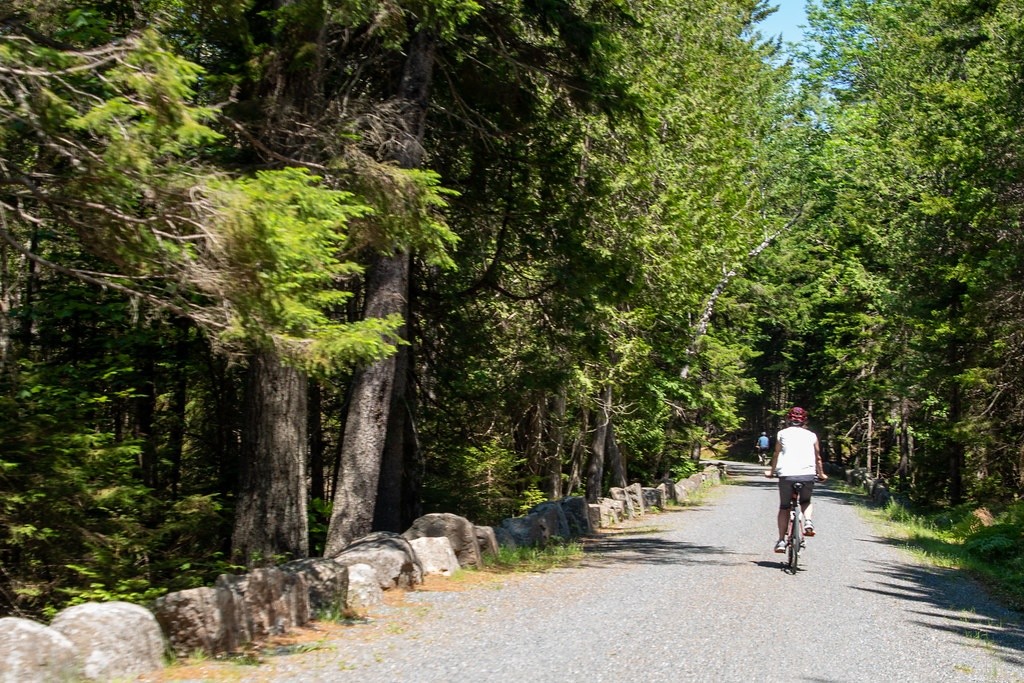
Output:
[758, 457, 763, 462]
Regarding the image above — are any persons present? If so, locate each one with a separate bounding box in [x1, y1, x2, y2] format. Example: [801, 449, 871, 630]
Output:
[757, 432, 769, 462]
[765, 407, 828, 552]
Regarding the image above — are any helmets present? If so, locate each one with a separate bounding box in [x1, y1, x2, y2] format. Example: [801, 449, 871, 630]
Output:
[784, 407, 808, 426]
[761, 432, 766, 435]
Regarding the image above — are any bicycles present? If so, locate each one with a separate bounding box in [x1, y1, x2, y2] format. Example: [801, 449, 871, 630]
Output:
[766, 472, 825, 574]
[755, 445, 770, 467]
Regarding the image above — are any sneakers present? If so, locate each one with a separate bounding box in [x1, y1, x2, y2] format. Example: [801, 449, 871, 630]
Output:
[802, 519, 815, 537]
[774, 540, 786, 553]
